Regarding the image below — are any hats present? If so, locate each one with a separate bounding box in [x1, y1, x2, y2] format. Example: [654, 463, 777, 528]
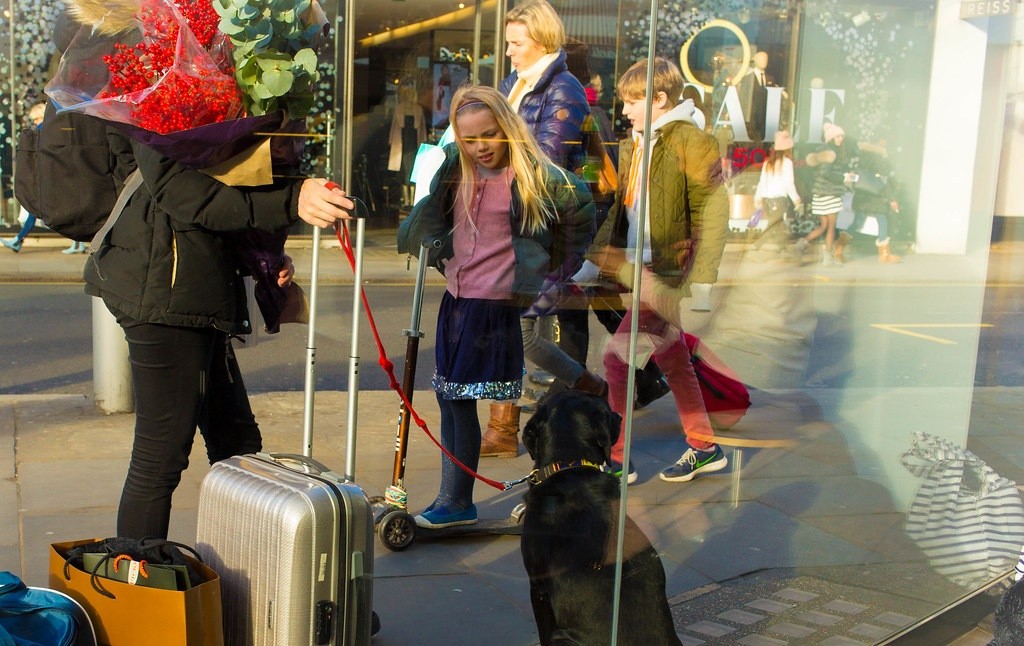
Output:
[564, 36, 591, 85]
[824, 123, 845, 142]
[774, 130, 793, 151]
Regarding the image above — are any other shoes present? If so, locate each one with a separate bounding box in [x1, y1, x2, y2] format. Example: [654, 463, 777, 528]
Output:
[415, 504, 478, 530]
[634, 377, 670, 410]
[521, 403, 538, 414]
[529, 367, 556, 386]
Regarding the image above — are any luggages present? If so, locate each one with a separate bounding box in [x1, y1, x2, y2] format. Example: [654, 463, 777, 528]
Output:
[197, 197, 375, 646]
[683, 332, 751, 430]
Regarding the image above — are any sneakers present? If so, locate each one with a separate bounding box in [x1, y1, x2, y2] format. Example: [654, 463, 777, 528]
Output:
[660, 443, 728, 482]
[611, 460, 638, 483]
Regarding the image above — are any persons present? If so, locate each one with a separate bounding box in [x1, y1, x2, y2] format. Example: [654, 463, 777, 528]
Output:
[835, 128, 903, 266]
[0, 0, 353, 540]
[598, 56, 729, 485]
[746, 130, 801, 266]
[387, 84, 427, 210]
[476, 0, 673, 460]
[396, 83, 596, 526]
[788, 123, 851, 269]
[738, 52, 775, 140]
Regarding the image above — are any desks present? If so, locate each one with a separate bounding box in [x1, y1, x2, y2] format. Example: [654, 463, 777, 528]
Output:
[684, 429, 808, 510]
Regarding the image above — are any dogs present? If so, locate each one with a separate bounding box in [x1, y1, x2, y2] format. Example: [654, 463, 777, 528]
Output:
[521, 388, 683, 646]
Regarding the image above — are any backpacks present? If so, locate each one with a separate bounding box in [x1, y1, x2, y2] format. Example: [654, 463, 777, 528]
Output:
[0, 36, 142, 254]
[0, 571, 97, 646]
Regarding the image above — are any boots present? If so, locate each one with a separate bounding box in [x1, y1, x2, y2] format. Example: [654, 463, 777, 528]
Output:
[832, 231, 852, 264]
[562, 362, 608, 398]
[788, 237, 810, 266]
[479, 403, 521, 458]
[875, 236, 901, 263]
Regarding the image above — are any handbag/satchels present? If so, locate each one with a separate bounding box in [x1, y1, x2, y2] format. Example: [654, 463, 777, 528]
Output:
[586, 116, 617, 202]
[747, 209, 763, 228]
[898, 432, 1023, 595]
[49, 535, 225, 646]
[855, 169, 888, 195]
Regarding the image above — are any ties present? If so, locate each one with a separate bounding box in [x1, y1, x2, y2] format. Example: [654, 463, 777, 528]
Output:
[761, 73, 764, 87]
